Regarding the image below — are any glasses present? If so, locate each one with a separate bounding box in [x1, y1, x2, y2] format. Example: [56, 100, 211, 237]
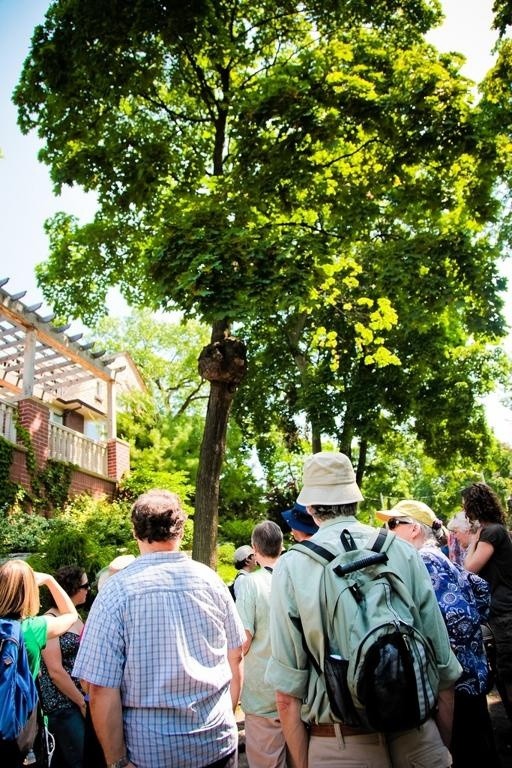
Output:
[77, 583, 90, 589]
[386, 520, 413, 531]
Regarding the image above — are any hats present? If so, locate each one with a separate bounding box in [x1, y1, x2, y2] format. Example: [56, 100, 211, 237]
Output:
[296, 451, 365, 505]
[378, 501, 434, 527]
[282, 505, 316, 537]
[233, 545, 255, 560]
[97, 554, 136, 591]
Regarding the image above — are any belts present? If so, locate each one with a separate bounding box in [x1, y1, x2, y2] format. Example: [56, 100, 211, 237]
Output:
[308, 723, 380, 737]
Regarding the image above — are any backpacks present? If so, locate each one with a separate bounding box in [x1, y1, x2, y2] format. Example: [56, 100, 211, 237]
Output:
[0, 618, 40, 768]
[228, 571, 247, 604]
[288, 526, 442, 738]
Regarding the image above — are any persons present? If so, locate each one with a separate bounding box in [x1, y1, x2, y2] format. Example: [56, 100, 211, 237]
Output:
[280, 503, 320, 542]
[263, 450, 462, 768]
[0, 558, 79, 768]
[231, 545, 257, 606]
[446, 510, 480, 567]
[375, 499, 502, 768]
[37, 565, 107, 768]
[460, 482, 512, 727]
[234, 520, 288, 768]
[70, 489, 248, 768]
